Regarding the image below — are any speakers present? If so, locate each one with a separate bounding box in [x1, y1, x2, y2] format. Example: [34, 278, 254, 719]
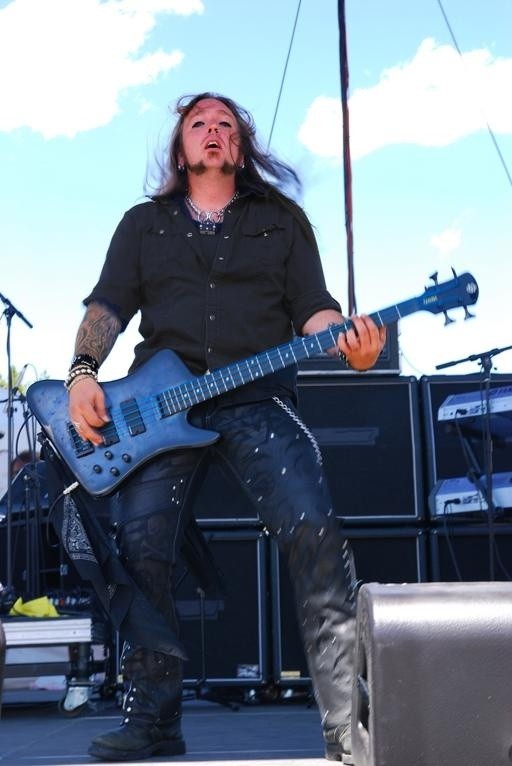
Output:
[348, 572, 512, 766]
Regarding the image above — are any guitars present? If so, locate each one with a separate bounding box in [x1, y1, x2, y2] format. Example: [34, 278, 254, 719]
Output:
[26, 266, 479, 498]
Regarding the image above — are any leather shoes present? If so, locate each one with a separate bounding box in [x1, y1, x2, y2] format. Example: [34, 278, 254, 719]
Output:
[87, 718, 185, 760]
[322, 719, 369, 765]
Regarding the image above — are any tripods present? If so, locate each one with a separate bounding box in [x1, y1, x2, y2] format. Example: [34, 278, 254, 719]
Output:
[181, 588, 239, 711]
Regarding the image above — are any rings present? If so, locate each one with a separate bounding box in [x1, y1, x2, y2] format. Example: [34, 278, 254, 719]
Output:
[72, 421, 82, 432]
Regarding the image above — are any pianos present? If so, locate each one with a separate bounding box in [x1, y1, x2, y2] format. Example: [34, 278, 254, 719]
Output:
[428, 383, 509, 517]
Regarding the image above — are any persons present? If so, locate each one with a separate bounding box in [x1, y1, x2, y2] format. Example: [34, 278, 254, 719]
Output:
[60, 88, 389, 765]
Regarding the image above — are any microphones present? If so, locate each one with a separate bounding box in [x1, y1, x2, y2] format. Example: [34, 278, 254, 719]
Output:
[0, 293, 33, 329]
[3, 365, 28, 412]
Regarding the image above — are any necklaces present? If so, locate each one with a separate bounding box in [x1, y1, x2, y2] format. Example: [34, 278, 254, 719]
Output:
[183, 190, 241, 236]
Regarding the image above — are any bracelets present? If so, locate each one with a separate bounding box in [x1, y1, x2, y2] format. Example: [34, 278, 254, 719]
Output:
[62, 353, 100, 391]
